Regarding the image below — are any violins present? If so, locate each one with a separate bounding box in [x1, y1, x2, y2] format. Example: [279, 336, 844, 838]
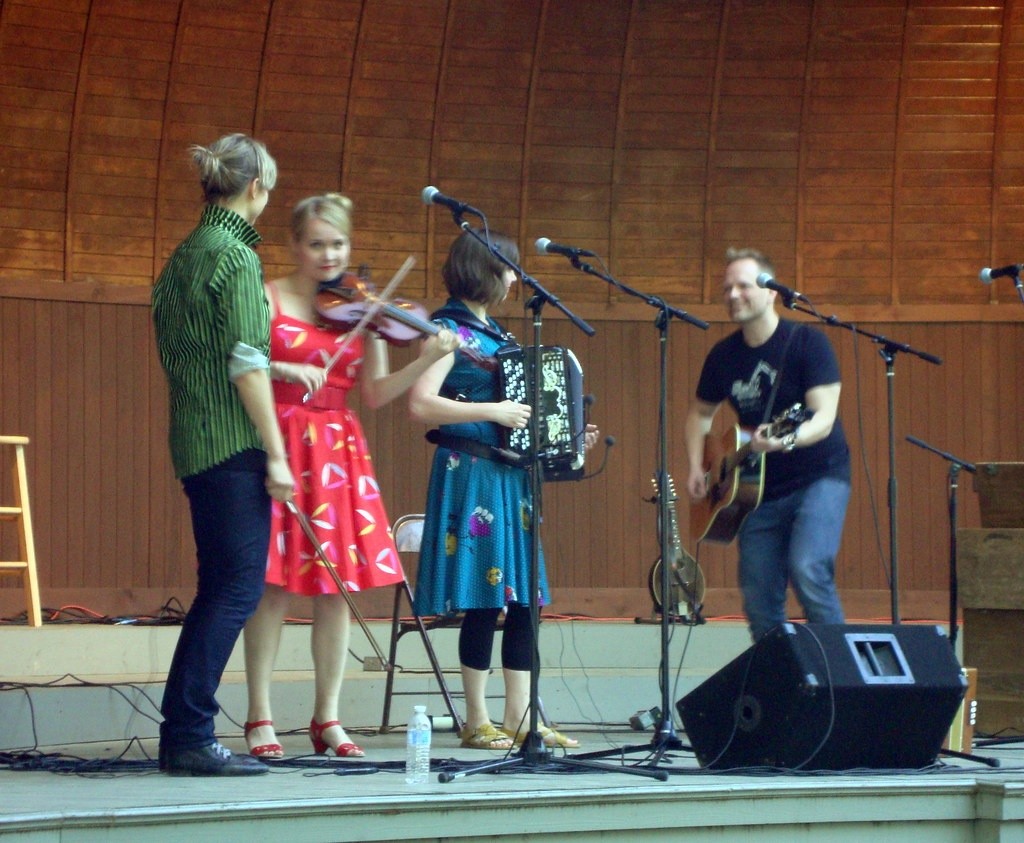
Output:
[308, 271, 501, 374]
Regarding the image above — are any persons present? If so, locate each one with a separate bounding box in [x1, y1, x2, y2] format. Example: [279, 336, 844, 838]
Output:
[417, 225, 599, 747]
[243, 186, 465, 755]
[148, 129, 298, 776]
[680, 248, 851, 645]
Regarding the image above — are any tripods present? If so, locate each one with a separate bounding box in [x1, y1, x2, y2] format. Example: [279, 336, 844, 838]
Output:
[434, 223, 669, 782]
[555, 259, 695, 768]
[789, 304, 1003, 770]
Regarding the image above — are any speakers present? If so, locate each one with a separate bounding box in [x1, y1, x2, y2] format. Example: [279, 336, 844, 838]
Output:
[674, 625, 969, 772]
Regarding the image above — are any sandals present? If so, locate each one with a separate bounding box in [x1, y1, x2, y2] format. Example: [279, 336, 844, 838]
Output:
[460, 724, 519, 750]
[500, 722, 581, 748]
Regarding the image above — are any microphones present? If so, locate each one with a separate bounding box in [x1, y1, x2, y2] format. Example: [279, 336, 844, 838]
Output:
[756, 273, 808, 301]
[979, 264, 1024, 284]
[421, 185, 485, 218]
[535, 237, 594, 257]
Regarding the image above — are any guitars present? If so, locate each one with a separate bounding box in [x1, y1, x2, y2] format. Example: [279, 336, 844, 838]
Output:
[648, 473, 706, 616]
[689, 401, 816, 546]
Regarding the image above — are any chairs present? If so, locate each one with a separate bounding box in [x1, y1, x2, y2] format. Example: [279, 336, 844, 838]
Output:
[381, 513, 552, 744]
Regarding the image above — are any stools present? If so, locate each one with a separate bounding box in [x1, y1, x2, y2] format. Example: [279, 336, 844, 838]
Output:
[1, 435, 43, 626]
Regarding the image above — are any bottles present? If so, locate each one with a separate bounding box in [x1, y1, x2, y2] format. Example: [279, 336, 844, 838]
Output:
[405, 706, 432, 785]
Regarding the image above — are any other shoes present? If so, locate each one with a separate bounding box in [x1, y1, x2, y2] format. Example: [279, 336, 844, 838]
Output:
[157, 739, 272, 777]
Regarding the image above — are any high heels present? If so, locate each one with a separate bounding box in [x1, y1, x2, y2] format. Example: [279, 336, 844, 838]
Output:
[244, 720, 284, 758]
[309, 718, 366, 757]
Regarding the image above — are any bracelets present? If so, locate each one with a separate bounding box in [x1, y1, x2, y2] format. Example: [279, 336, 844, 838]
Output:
[782, 431, 799, 453]
